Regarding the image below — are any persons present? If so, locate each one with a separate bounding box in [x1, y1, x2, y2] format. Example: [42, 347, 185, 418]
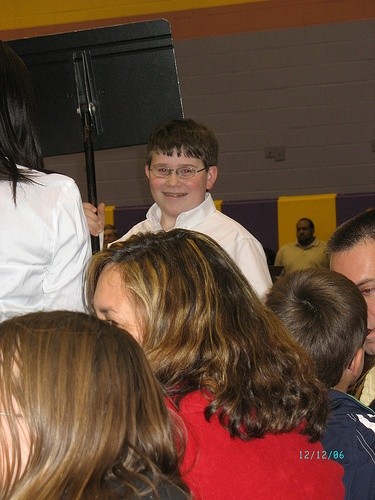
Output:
[84, 229, 346, 500]
[266, 269, 375, 500]
[273, 218, 329, 277]
[327, 209, 375, 412]
[0, 40, 104, 322]
[0, 311, 192, 500]
[108, 119, 271, 299]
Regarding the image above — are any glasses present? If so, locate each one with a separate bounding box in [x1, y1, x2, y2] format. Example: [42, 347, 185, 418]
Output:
[149, 163, 208, 179]
